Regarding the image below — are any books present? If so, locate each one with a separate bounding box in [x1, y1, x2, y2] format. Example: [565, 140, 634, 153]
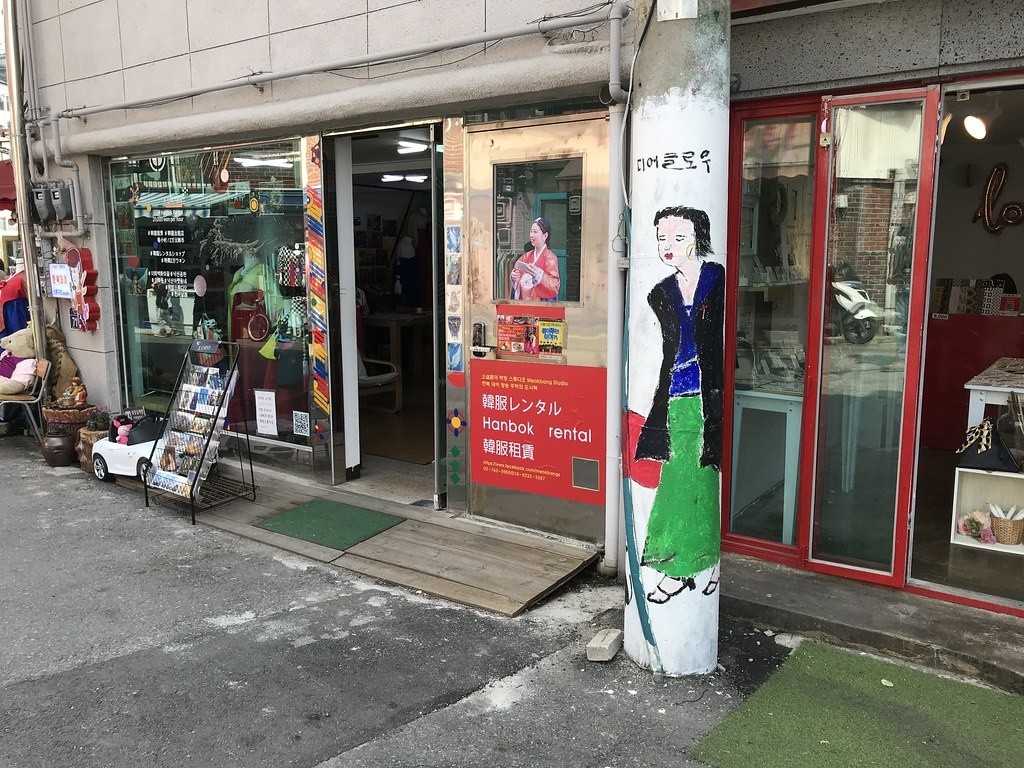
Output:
[152, 364, 237, 498]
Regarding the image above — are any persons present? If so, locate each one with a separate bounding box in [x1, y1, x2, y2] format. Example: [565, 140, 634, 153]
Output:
[68, 377, 86, 403]
[510, 217, 561, 302]
[151, 278, 179, 337]
[0, 259, 31, 356]
[226, 253, 308, 436]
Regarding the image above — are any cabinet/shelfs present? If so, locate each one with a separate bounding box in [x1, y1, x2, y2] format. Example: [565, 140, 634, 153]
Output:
[145, 339, 255, 526]
[950, 466, 1024, 555]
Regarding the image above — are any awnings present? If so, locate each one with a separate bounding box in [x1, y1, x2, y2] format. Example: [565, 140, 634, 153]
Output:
[0, 159, 17, 212]
[134, 191, 250, 219]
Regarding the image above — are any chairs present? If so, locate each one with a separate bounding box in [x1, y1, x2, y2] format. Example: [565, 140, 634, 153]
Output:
[1, 358, 52, 444]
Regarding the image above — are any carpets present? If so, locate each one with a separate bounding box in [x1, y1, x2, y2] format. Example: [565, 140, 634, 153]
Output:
[254, 497, 406, 551]
[689, 640, 1024, 768]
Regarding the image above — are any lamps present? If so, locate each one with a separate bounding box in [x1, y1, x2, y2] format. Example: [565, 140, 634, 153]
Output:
[964, 94, 1002, 138]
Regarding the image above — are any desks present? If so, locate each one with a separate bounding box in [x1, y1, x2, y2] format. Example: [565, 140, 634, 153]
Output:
[361, 311, 433, 414]
[964, 356, 1024, 433]
[731, 368, 901, 545]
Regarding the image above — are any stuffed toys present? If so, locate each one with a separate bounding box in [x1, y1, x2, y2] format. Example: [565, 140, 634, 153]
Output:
[0, 324, 37, 394]
[113, 420, 132, 444]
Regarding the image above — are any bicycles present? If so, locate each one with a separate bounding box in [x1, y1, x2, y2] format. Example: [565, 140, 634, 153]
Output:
[247, 299, 270, 343]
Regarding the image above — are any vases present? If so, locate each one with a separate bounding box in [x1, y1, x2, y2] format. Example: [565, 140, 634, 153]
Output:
[95, 422, 110, 431]
[42, 432, 77, 466]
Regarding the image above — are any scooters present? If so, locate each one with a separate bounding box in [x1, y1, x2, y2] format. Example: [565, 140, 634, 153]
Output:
[829, 278, 879, 345]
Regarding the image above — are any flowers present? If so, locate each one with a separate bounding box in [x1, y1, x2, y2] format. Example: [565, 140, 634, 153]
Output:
[47, 418, 69, 435]
[86, 406, 112, 422]
[958, 514, 998, 545]
